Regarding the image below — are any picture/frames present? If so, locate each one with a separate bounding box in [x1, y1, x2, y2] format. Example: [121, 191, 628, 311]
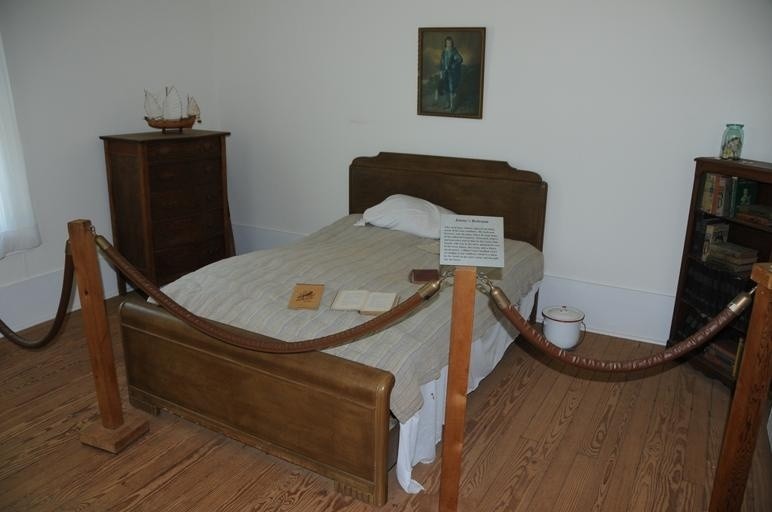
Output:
[415, 25, 488, 119]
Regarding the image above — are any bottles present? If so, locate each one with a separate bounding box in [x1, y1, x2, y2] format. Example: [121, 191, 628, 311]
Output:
[719, 123, 745, 160]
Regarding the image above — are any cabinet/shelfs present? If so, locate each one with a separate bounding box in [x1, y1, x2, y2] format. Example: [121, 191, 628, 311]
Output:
[100, 127, 236, 299]
[662, 154, 772, 392]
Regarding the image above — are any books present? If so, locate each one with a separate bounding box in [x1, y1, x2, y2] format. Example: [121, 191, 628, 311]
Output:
[674, 168, 771, 371]
[411, 268, 440, 284]
[285, 279, 324, 311]
[330, 287, 402, 316]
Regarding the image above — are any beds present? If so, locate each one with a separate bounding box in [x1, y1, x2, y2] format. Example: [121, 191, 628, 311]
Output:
[115, 151, 548, 504]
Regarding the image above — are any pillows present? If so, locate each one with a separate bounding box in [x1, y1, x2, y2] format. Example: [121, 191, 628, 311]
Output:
[350, 191, 462, 240]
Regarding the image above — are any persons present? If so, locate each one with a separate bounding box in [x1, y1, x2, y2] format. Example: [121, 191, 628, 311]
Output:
[431, 36, 463, 112]
